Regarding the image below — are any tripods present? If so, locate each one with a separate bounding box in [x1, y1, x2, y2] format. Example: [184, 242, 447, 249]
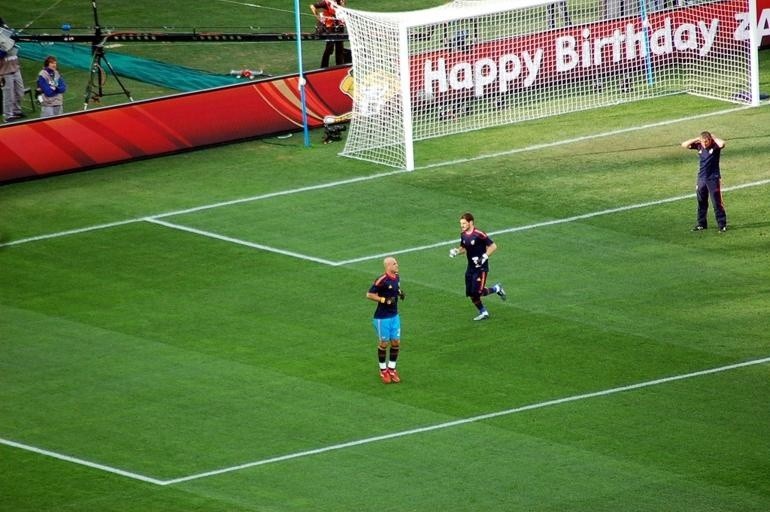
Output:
[82, 37, 133, 111]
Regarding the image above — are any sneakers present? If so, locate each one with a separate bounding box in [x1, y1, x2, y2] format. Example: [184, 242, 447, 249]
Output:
[495, 283, 506, 301]
[691, 224, 707, 232]
[474, 311, 490, 321]
[718, 225, 726, 234]
[389, 368, 400, 384]
[380, 369, 391, 384]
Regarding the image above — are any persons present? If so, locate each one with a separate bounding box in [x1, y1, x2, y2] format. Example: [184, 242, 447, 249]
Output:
[310, 0, 345, 69]
[35, 55, 66, 118]
[0, 18, 29, 121]
[682, 129, 729, 234]
[447, 211, 506, 322]
[546, 0, 574, 32]
[365, 255, 407, 386]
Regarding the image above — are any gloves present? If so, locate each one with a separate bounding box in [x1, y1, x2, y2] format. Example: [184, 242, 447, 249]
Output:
[449, 247, 458, 258]
[400, 291, 405, 300]
[471, 253, 488, 268]
[380, 296, 396, 304]
